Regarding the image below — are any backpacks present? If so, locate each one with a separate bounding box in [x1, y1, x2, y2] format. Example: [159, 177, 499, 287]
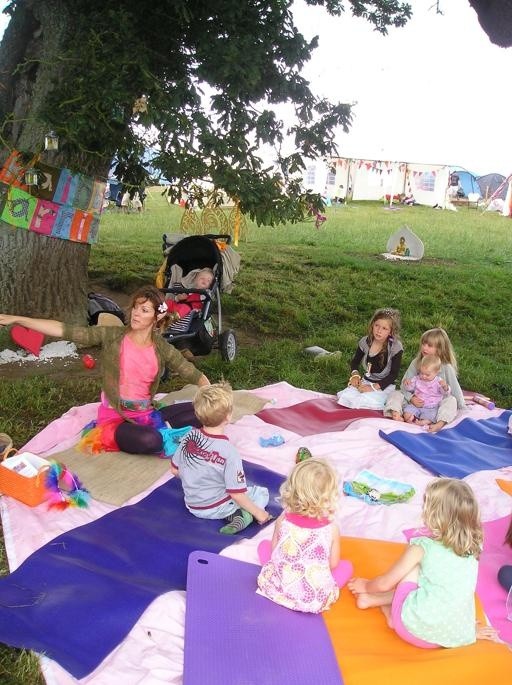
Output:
[87, 288, 125, 326]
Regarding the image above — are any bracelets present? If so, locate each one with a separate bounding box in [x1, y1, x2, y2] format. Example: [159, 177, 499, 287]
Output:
[371, 383, 378, 391]
[349, 375, 361, 381]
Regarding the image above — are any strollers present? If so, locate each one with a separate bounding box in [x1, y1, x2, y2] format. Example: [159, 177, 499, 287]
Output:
[156, 234, 237, 365]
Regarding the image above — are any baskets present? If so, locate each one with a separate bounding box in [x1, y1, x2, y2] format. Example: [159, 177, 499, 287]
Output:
[0, 431, 13, 459]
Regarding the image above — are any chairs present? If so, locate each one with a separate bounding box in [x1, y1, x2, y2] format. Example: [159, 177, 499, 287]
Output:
[468, 193, 480, 210]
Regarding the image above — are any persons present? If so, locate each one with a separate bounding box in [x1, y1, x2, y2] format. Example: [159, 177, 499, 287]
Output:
[170, 380, 271, 536]
[497, 520, 512, 621]
[447, 171, 461, 200]
[402, 355, 451, 426]
[254, 458, 353, 615]
[383, 328, 467, 432]
[321, 184, 353, 207]
[347, 477, 501, 649]
[336, 310, 404, 411]
[121, 189, 143, 215]
[0, 284, 211, 455]
[165, 267, 214, 322]
